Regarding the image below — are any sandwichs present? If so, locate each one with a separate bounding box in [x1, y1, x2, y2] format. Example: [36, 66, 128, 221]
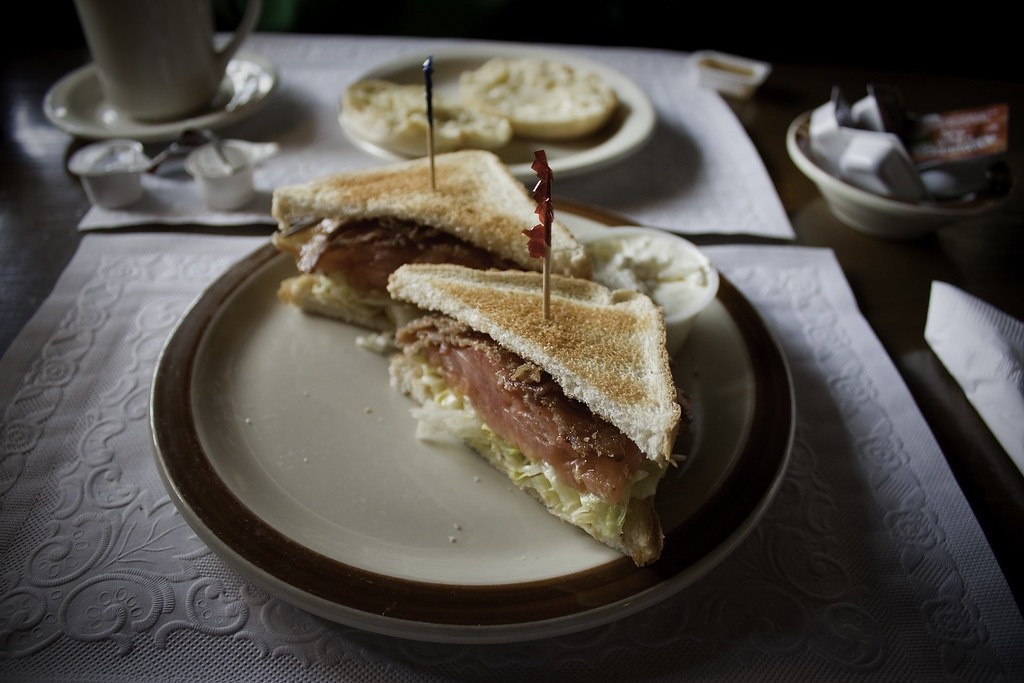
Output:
[384, 261, 682, 571]
[262, 145, 590, 335]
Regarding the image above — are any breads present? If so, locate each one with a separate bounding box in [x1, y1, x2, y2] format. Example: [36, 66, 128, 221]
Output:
[454, 53, 619, 141]
[339, 75, 516, 154]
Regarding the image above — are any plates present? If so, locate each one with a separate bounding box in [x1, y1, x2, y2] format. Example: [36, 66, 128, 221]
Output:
[147, 198, 795, 645]
[338, 51, 655, 182]
[42, 61, 278, 142]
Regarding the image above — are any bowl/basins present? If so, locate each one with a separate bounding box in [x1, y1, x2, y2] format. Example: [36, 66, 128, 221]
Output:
[788, 109, 1017, 237]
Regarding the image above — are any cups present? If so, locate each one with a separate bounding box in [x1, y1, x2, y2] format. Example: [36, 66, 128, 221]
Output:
[74, 0, 261, 121]
[186, 140, 257, 210]
[67, 138, 143, 206]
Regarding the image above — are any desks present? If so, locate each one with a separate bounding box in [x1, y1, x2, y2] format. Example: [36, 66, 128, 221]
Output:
[0, 33, 1024, 634]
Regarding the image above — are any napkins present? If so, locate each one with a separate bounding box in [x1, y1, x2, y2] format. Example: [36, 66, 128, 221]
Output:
[924, 281, 1024, 477]
